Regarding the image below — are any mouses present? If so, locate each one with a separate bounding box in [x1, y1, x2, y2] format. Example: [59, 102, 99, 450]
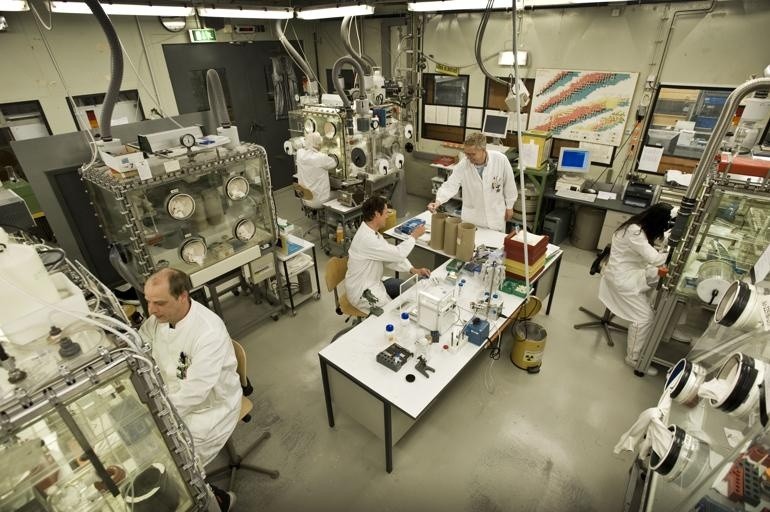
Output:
[588, 188, 596, 193]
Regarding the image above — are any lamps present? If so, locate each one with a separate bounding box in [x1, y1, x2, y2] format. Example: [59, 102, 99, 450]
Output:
[495, 49, 530, 67]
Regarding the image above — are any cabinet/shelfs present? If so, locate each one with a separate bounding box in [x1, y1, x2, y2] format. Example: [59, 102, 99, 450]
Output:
[428, 161, 465, 216]
[506, 163, 557, 237]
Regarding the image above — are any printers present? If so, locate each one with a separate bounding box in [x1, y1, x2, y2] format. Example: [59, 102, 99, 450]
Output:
[620, 179, 657, 209]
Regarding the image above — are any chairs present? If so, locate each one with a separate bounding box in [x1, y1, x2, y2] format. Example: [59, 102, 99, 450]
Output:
[289, 181, 336, 251]
[204, 337, 282, 492]
[323, 255, 384, 344]
[572, 240, 632, 347]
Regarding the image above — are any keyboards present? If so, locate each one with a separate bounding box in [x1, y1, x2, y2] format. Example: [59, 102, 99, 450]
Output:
[555, 189, 597, 204]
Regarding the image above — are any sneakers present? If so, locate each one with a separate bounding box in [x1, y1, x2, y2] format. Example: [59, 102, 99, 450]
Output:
[624, 355, 659, 378]
[218, 488, 238, 512]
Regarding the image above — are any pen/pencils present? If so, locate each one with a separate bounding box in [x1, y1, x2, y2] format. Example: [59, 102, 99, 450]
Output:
[451, 332, 453, 346]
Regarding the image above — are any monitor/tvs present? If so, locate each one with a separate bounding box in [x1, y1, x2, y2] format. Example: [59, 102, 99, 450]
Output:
[556, 146, 593, 180]
[481, 113, 510, 144]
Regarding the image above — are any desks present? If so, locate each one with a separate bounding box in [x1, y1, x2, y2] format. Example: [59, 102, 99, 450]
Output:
[317, 255, 537, 478]
[544, 179, 652, 254]
[319, 196, 362, 259]
[382, 205, 566, 323]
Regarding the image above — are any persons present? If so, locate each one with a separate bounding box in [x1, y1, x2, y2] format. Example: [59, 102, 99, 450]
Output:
[601, 200, 672, 375]
[426, 131, 519, 234]
[131, 265, 241, 510]
[296, 132, 343, 207]
[346, 197, 430, 320]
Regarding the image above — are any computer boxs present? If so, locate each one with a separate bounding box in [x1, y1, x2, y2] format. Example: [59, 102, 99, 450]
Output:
[554, 177, 586, 192]
[543, 208, 571, 245]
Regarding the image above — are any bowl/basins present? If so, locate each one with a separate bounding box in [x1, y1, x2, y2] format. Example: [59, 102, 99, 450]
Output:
[121, 464, 180, 511]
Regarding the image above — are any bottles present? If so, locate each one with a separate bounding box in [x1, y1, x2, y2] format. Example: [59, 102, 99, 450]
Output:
[483, 291, 499, 319]
[297, 271, 312, 293]
[473, 267, 480, 279]
[373, 71, 386, 104]
[49, 326, 62, 342]
[401, 313, 410, 333]
[385, 325, 396, 347]
[458, 278, 467, 295]
[336, 223, 344, 243]
[58, 337, 83, 362]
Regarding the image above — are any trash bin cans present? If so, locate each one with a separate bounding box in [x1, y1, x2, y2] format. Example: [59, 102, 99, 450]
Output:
[510, 296, 547, 373]
